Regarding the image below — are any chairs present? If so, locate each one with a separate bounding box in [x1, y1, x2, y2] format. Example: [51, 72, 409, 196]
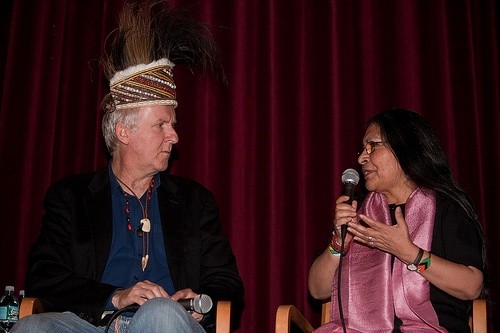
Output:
[17, 297, 232, 333]
[274, 295, 488, 333]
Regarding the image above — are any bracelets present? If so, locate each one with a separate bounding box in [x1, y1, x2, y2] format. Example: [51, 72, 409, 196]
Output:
[417, 253, 431, 274]
[329, 236, 349, 257]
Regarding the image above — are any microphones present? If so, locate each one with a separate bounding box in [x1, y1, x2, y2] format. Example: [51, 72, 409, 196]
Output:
[341, 168, 360, 239]
[125, 293, 214, 314]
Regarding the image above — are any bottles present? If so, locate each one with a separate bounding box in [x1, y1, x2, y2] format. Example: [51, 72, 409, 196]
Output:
[0, 286, 19, 333]
[18, 290, 24, 304]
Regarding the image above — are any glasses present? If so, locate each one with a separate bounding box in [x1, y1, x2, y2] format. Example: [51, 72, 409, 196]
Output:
[358, 141, 386, 155]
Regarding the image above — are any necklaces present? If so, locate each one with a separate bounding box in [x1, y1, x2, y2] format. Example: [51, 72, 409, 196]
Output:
[116, 176, 154, 272]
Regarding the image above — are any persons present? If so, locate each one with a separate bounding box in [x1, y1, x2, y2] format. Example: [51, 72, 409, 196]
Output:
[9, 104, 245, 333]
[308, 108, 484, 333]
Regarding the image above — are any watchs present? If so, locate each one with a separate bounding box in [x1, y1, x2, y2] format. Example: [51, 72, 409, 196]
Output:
[408, 248, 424, 271]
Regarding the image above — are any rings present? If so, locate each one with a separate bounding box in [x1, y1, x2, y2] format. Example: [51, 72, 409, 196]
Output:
[369, 237, 372, 241]
[369, 242, 374, 247]
